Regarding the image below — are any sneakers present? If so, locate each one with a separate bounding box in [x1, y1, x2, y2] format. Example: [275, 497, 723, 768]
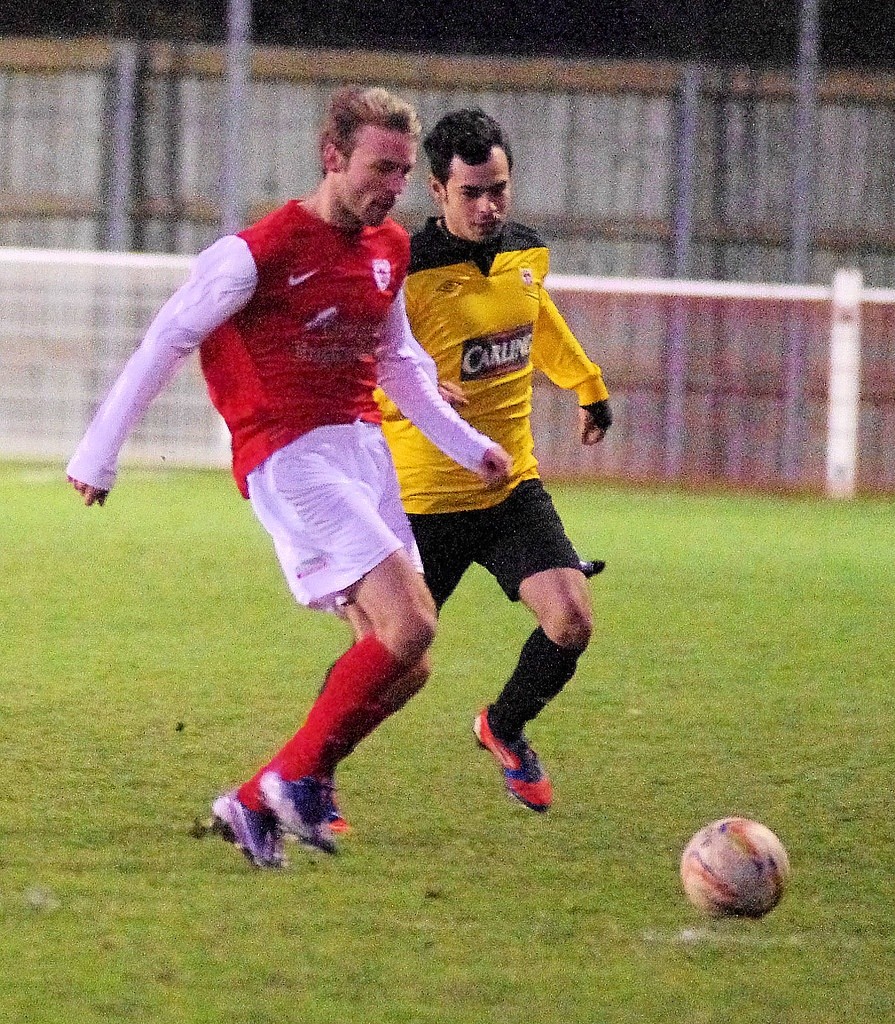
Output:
[472, 702, 553, 811]
[253, 772, 337, 857]
[319, 777, 349, 833]
[212, 787, 289, 868]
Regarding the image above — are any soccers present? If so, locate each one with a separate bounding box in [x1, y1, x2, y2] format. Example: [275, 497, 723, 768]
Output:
[681, 816, 791, 921]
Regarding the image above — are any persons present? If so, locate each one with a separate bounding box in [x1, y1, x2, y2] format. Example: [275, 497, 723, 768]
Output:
[64, 87, 516, 869]
[314, 101, 614, 832]
